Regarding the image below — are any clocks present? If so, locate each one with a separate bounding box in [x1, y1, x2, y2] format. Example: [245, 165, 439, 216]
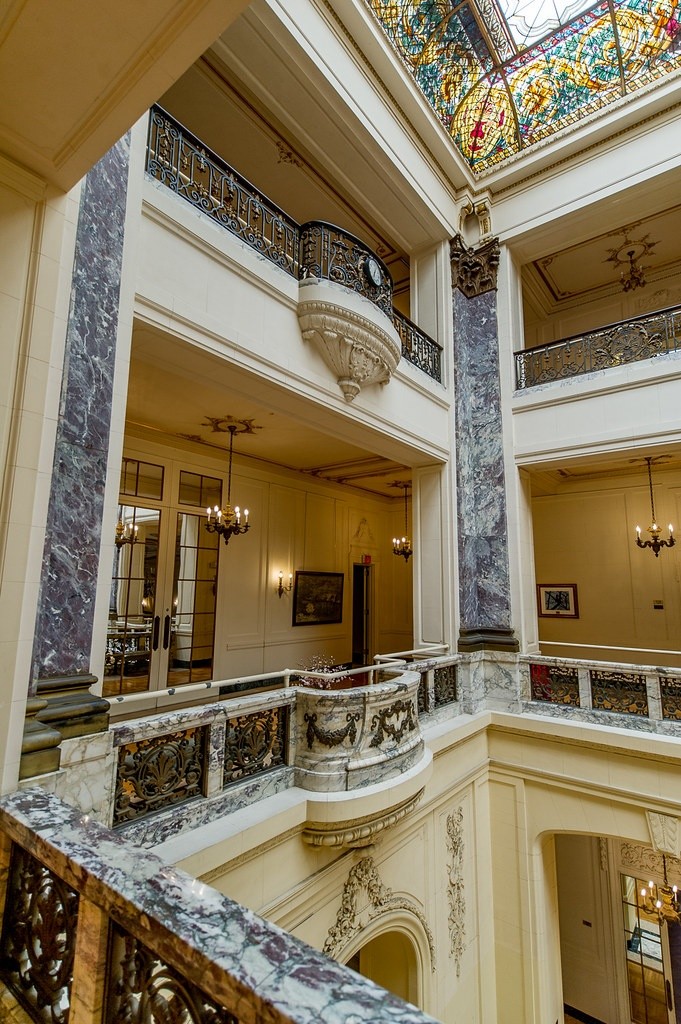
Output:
[366, 259, 381, 287]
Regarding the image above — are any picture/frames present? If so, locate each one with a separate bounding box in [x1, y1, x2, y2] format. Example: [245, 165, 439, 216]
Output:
[292, 571, 344, 626]
[536, 583, 579, 619]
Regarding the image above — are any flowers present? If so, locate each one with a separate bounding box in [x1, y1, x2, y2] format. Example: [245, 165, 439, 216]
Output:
[298, 651, 354, 690]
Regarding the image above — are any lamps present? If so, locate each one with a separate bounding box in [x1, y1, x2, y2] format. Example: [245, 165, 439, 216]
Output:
[205, 426, 251, 545]
[621, 251, 647, 293]
[641, 853, 681, 927]
[275, 571, 293, 599]
[634, 458, 677, 558]
[391, 483, 412, 562]
[115, 505, 139, 554]
